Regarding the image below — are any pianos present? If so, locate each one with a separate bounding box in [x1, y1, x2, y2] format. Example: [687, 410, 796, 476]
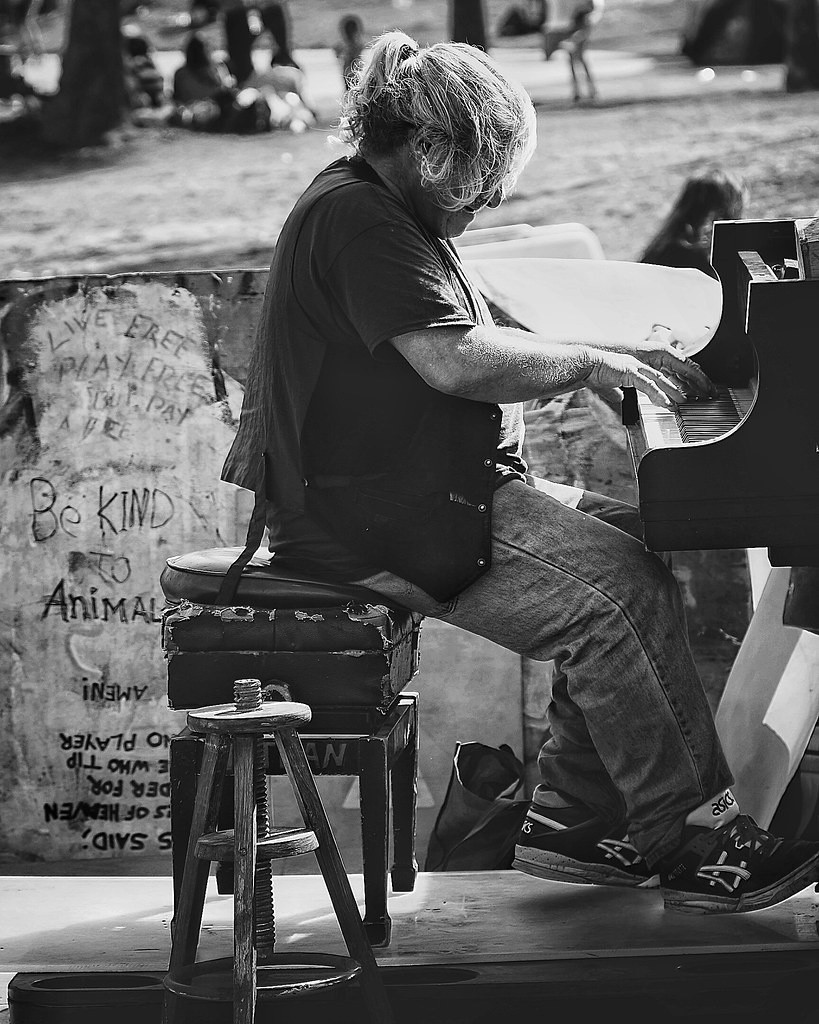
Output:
[600, 215, 819, 838]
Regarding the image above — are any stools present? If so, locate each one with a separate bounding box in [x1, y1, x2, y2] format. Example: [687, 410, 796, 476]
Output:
[158, 543, 422, 947]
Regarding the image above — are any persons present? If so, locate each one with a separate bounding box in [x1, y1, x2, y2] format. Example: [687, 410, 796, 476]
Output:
[220, 30, 818, 914]
[335, 14, 369, 91]
[123, 0, 304, 134]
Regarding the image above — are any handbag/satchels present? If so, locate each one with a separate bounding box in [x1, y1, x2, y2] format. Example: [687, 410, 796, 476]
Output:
[424, 740, 531, 872]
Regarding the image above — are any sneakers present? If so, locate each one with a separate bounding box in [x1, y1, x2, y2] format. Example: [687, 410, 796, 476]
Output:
[659, 814, 819, 913]
[513, 803, 661, 888]
